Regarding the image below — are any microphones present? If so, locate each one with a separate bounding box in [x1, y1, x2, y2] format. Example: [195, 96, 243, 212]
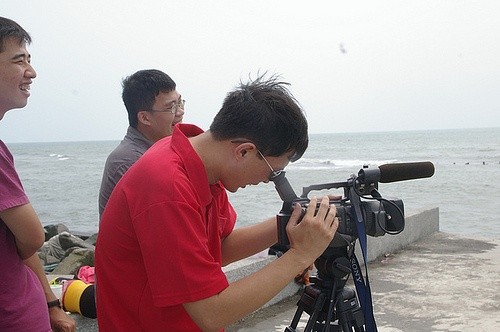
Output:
[359, 162, 434, 184]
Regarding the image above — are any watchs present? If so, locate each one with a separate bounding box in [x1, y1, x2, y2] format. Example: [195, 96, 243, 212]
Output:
[47, 298, 61, 309]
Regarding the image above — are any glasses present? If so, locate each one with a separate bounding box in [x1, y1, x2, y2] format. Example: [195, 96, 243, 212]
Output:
[140, 99, 186, 113]
[231, 139, 286, 181]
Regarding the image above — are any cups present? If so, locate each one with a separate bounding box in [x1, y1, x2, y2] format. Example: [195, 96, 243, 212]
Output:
[50, 284, 62, 311]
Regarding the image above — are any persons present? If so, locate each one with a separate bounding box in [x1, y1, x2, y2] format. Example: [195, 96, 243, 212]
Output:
[99, 69, 186, 222]
[0, 17, 75, 332]
[94, 68, 339, 332]
